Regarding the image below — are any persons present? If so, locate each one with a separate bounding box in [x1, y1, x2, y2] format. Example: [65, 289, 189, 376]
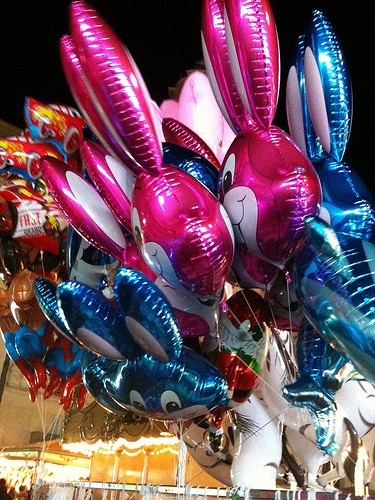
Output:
[0, 478, 35, 500]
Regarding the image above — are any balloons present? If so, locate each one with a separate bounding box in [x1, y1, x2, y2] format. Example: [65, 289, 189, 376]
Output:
[0, 3, 373, 489]
